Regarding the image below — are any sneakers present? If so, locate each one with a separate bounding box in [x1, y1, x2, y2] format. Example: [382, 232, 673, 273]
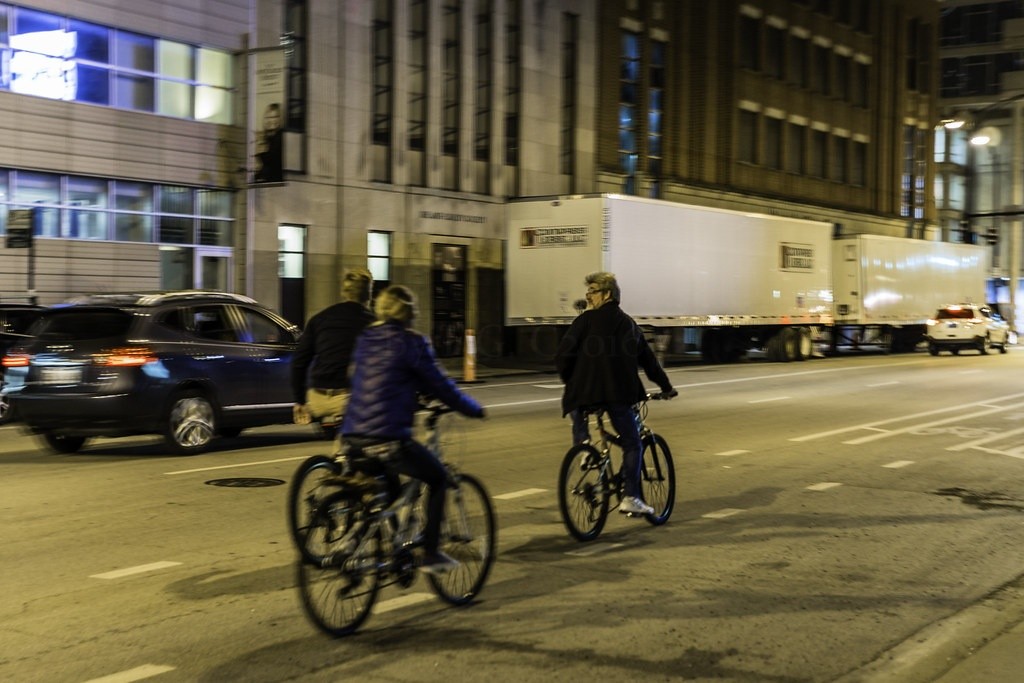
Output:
[420, 552, 459, 574]
[580, 453, 592, 471]
[618, 496, 656, 514]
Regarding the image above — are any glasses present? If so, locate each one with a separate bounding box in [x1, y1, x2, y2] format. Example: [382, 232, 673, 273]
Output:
[585, 289, 604, 294]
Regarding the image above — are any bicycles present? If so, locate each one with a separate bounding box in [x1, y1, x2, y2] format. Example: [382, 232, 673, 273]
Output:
[296, 404, 497, 635]
[289, 391, 447, 567]
[556, 391, 676, 542]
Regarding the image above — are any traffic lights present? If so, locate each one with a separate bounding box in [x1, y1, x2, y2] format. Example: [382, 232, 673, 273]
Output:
[986, 226, 1000, 246]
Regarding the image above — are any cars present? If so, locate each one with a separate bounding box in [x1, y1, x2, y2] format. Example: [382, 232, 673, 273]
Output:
[4, 289, 308, 455]
[0, 303, 54, 427]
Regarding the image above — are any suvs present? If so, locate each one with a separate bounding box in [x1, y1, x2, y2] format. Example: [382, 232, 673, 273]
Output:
[928, 303, 1011, 356]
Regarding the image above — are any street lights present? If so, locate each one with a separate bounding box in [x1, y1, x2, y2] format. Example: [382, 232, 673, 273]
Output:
[926, 92, 1024, 246]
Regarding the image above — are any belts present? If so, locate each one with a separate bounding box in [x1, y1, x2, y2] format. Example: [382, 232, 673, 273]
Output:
[315, 388, 352, 396]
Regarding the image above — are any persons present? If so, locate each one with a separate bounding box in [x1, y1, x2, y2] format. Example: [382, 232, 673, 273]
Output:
[254, 103, 283, 182]
[558, 272, 677, 514]
[290, 267, 488, 574]
